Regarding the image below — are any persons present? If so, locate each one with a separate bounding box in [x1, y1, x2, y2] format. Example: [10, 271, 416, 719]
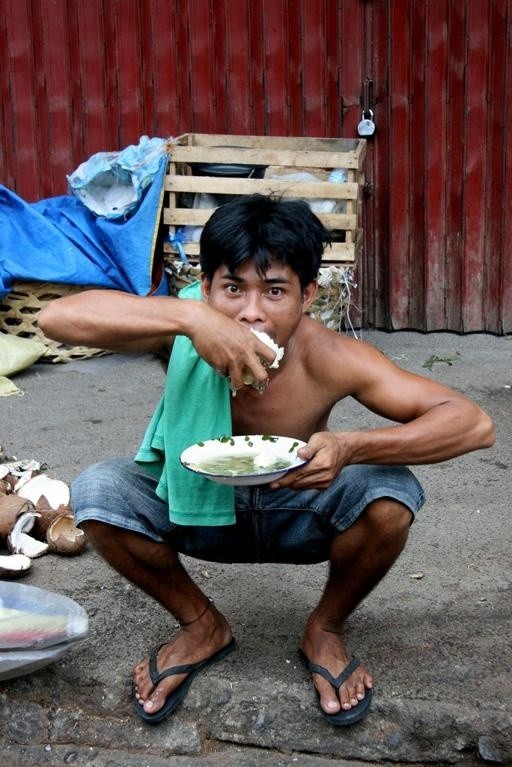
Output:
[38, 195, 496, 727]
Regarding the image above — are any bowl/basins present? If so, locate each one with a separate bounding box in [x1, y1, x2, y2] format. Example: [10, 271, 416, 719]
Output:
[185, 145, 269, 198]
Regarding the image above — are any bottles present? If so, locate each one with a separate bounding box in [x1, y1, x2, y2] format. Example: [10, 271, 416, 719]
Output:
[328, 167, 348, 182]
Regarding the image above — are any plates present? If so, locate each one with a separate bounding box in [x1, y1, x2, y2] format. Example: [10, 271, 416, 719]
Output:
[179, 431, 309, 487]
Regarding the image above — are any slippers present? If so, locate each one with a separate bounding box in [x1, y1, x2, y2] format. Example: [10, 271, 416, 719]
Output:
[299, 634, 373, 728]
[134, 636, 235, 722]
